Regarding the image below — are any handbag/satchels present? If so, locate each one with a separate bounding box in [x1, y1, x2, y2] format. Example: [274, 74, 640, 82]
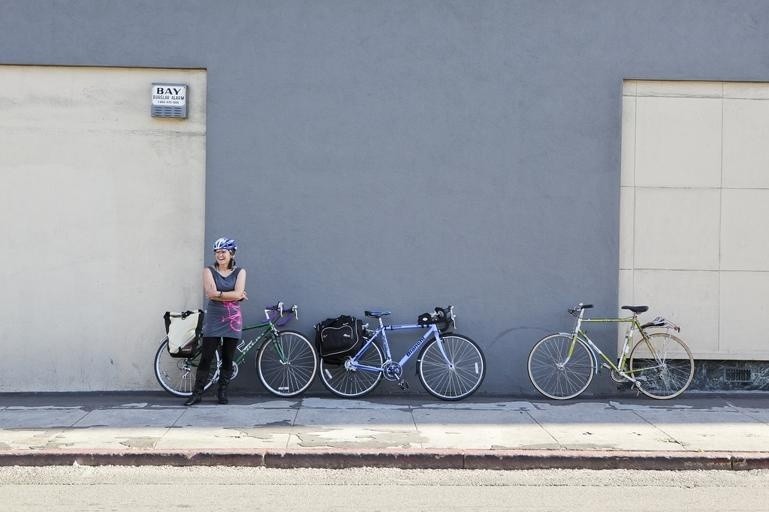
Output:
[164, 309, 204, 357]
[315, 314, 362, 365]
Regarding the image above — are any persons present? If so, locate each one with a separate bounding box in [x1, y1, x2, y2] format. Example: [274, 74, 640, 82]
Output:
[183, 237, 248, 405]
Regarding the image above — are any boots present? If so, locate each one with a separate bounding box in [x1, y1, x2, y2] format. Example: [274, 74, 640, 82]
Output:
[218, 367, 234, 404]
[184, 370, 209, 405]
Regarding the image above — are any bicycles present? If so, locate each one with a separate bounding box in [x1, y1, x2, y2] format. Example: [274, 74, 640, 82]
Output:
[153, 303, 318, 399]
[527, 305, 694, 400]
[319, 306, 487, 401]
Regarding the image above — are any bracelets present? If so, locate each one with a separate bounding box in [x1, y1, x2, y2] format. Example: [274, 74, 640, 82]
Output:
[218, 291, 222, 298]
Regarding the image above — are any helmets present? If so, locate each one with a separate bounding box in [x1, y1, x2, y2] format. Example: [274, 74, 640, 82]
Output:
[213, 237, 238, 252]
